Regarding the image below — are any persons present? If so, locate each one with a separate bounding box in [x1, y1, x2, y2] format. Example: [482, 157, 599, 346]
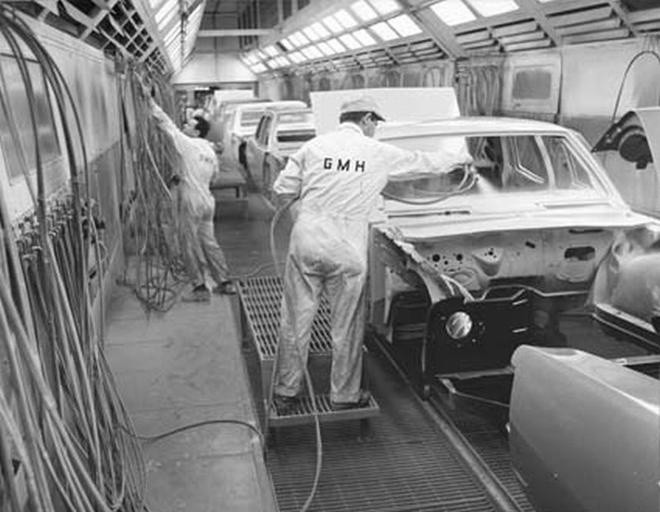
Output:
[272, 99, 474, 410]
[134, 70, 237, 302]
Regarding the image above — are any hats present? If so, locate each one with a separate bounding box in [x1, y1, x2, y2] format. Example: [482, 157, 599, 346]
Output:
[341, 97, 386, 122]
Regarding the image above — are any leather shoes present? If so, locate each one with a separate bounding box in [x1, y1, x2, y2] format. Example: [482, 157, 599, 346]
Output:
[330, 390, 371, 410]
[275, 394, 296, 408]
[180, 288, 210, 302]
[212, 283, 236, 294]
